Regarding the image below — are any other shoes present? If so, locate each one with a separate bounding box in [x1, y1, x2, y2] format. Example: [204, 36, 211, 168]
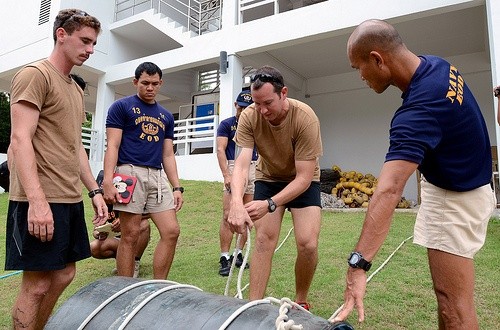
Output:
[112, 267, 117, 273]
[133, 260, 139, 278]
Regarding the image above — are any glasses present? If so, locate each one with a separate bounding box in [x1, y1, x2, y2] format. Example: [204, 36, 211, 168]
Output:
[60, 11, 89, 28]
[249, 73, 282, 83]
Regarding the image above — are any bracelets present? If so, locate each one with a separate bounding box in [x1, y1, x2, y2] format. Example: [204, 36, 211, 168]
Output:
[173, 187, 184, 193]
[89, 188, 104, 198]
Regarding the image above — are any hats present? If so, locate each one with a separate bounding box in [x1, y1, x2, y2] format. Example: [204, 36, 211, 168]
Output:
[236, 90, 253, 107]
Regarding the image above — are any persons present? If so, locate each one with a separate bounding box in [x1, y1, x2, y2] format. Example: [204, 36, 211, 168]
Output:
[102, 62, 183, 282]
[228, 66, 324, 312]
[217, 89, 258, 277]
[5, 10, 109, 330]
[331, 19, 497, 330]
[90, 170, 150, 278]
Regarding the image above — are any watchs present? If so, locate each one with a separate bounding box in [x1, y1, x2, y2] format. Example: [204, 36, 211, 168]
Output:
[267, 199, 276, 212]
[347, 252, 372, 272]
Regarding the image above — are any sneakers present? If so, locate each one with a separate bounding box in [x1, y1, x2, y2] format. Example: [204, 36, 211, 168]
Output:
[218, 255, 232, 277]
[232, 253, 249, 269]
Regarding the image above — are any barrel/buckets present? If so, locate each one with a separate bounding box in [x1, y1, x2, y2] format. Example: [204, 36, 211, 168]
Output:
[43, 276, 353, 330]
